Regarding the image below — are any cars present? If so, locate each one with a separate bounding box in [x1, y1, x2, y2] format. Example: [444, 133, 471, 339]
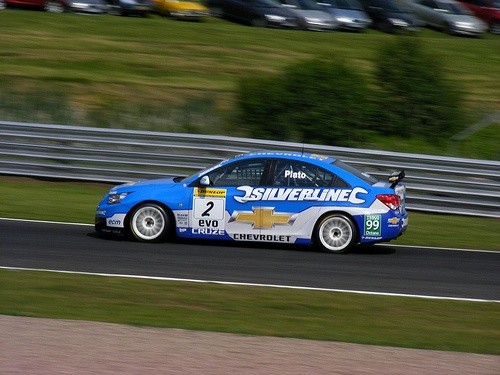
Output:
[95, 147, 409, 255]
[2, 1, 113, 15]
[150, 1, 211, 21]
[360, 0, 424, 37]
[407, 0, 488, 37]
[205, 1, 309, 31]
[279, 0, 339, 34]
[458, 0, 500, 35]
[322, 0, 371, 32]
